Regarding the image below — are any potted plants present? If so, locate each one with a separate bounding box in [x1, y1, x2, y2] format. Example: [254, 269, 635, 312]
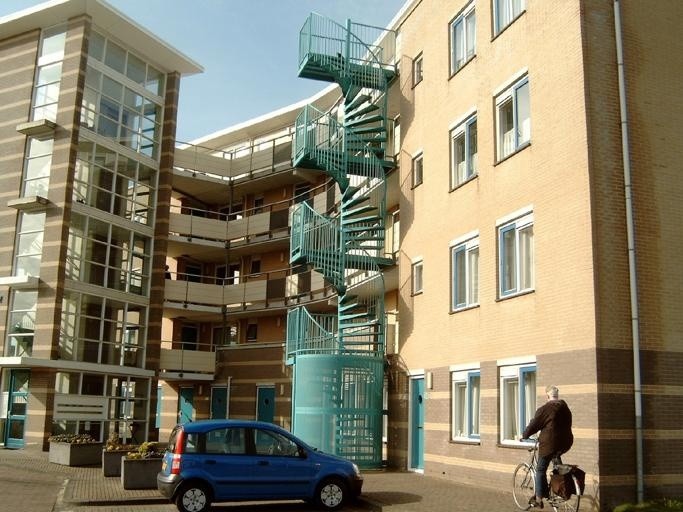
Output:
[48, 431, 164, 490]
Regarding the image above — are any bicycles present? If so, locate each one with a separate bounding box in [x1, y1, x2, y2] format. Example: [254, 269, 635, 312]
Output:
[509, 435, 582, 511]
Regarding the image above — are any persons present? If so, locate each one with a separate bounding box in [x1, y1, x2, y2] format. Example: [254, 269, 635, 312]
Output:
[521, 384, 574, 508]
[165, 264, 172, 280]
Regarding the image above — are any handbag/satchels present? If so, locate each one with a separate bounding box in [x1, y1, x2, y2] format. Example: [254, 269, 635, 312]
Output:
[551, 464, 585, 501]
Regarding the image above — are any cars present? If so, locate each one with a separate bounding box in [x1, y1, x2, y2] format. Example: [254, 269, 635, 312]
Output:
[154, 417, 362, 511]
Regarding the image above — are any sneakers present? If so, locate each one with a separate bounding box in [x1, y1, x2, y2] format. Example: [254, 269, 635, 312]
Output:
[529, 500, 543, 509]
